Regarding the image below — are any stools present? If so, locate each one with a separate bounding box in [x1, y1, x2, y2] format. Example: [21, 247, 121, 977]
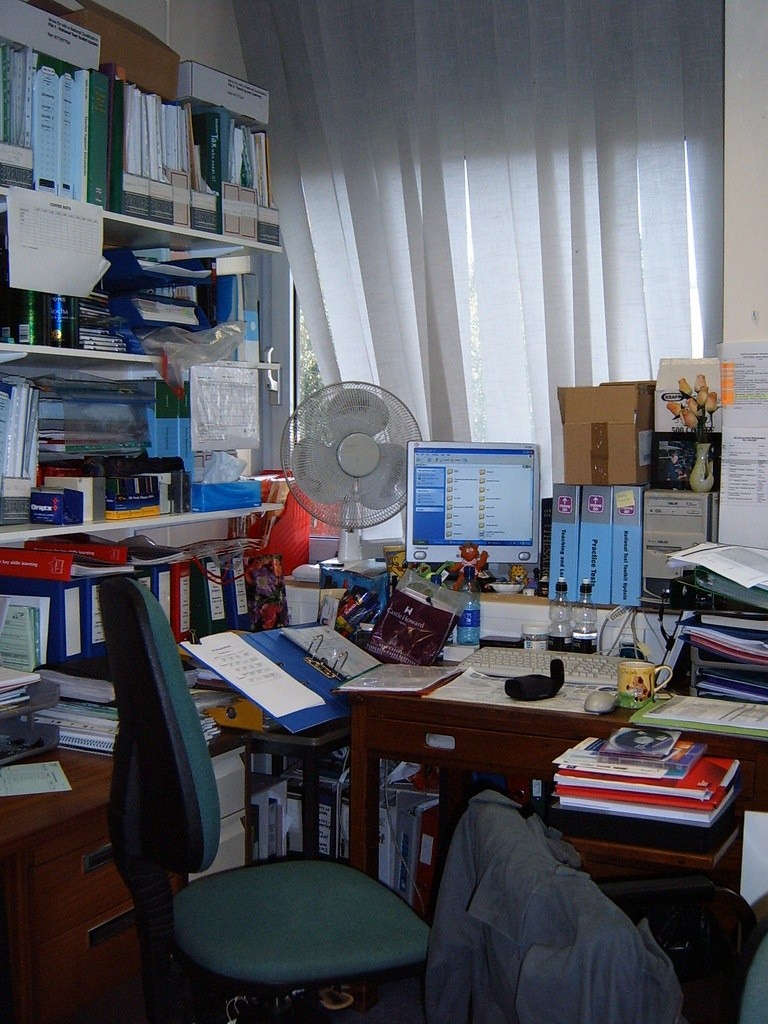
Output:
[241, 720, 350, 863]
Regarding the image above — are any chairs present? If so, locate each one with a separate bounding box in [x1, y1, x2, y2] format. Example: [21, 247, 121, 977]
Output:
[96, 572, 432, 1024]
[424, 789, 755, 1024]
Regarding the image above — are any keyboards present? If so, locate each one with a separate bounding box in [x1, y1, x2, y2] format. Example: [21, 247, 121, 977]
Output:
[457, 647, 633, 687]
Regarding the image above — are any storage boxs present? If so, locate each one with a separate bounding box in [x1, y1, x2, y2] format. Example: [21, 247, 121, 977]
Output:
[44, 475, 106, 521]
[0, 0, 100, 71]
[24, 0, 180, 100]
[31, 486, 84, 525]
[556, 380, 656, 485]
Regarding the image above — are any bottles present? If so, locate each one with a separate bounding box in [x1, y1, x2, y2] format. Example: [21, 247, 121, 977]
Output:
[457, 566, 481, 645]
[548, 577, 598, 654]
[430, 575, 453, 645]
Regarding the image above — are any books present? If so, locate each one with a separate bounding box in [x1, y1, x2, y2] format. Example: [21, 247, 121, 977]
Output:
[0, 371, 40, 484]
[553, 736, 745, 828]
[0, 666, 222, 756]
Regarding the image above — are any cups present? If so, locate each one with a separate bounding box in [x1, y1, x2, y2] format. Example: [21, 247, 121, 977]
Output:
[383, 546, 413, 597]
[521, 624, 548, 650]
[617, 661, 673, 709]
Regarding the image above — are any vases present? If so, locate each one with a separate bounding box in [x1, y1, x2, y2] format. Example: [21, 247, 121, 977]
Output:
[689, 443, 714, 492]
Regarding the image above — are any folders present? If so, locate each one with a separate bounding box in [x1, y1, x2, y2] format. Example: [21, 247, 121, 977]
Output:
[693, 566, 768, 607]
[675, 618, 768, 667]
[692, 669, 768, 705]
[178, 620, 381, 733]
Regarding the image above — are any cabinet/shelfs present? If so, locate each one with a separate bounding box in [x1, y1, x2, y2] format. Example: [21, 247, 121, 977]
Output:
[0, 187, 295, 541]
[177, 60, 270, 126]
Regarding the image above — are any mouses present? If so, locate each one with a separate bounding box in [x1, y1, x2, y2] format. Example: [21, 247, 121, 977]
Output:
[583, 691, 620, 712]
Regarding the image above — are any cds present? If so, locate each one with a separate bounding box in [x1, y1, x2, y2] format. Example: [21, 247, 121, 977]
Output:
[610, 730, 673, 753]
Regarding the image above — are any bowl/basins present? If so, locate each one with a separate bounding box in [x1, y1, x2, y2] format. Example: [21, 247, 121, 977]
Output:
[489, 583, 524, 594]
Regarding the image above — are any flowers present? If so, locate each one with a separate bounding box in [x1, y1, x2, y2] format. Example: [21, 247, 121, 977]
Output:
[667, 374, 722, 444]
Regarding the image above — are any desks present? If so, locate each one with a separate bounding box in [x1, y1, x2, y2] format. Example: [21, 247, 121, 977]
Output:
[348, 660, 768, 873]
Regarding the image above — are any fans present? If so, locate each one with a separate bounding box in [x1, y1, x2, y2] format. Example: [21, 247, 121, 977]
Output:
[280, 381, 422, 583]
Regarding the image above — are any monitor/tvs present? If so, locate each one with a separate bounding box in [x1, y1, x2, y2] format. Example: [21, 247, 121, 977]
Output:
[406, 441, 540, 565]
